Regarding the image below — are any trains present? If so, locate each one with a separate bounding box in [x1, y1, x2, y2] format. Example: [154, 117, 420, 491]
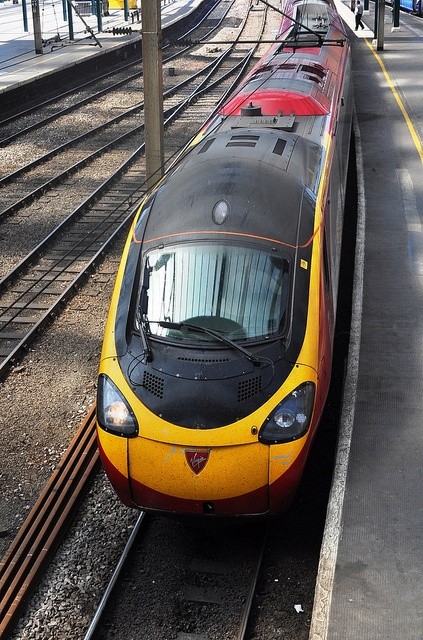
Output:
[97, 1, 350, 520]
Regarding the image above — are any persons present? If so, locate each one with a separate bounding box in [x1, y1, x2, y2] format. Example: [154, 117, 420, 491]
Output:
[353, 1, 364, 30]
